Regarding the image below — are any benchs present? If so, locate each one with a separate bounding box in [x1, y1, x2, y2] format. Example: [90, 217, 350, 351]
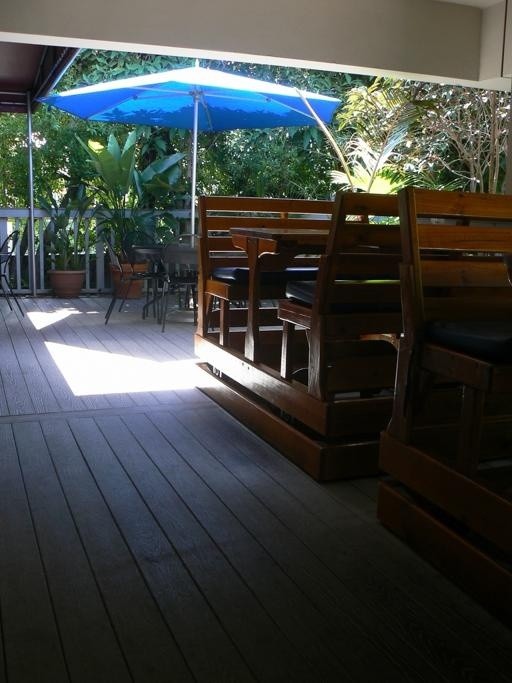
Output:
[375, 188, 511, 621]
[195, 188, 400, 484]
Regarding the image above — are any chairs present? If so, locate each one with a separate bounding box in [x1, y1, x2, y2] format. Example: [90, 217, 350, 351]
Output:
[1, 231, 26, 318]
[104, 231, 200, 332]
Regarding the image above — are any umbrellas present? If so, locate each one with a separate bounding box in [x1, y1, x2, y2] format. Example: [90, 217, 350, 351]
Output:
[28, 53, 346, 249]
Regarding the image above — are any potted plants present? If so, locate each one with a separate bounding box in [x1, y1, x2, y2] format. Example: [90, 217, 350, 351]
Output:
[43, 129, 185, 300]
